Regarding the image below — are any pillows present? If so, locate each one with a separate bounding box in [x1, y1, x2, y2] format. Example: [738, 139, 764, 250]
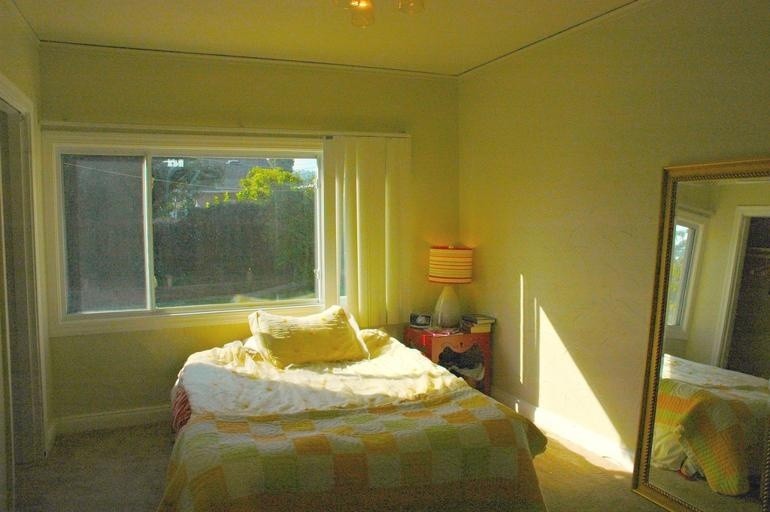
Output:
[247, 304, 371, 371]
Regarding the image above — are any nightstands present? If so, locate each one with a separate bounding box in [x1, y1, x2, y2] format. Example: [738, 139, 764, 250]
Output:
[404, 326, 492, 398]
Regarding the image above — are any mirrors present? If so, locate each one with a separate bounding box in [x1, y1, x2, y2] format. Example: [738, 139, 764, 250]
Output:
[628, 157, 769, 512]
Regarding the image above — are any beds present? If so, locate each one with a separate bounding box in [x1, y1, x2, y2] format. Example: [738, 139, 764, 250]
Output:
[170, 327, 529, 511]
[648, 353, 769, 494]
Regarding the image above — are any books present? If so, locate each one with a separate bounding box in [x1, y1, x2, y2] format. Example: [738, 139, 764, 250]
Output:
[459, 313, 496, 333]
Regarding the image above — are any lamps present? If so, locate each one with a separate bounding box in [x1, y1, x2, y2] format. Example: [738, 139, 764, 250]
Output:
[426, 243, 473, 327]
